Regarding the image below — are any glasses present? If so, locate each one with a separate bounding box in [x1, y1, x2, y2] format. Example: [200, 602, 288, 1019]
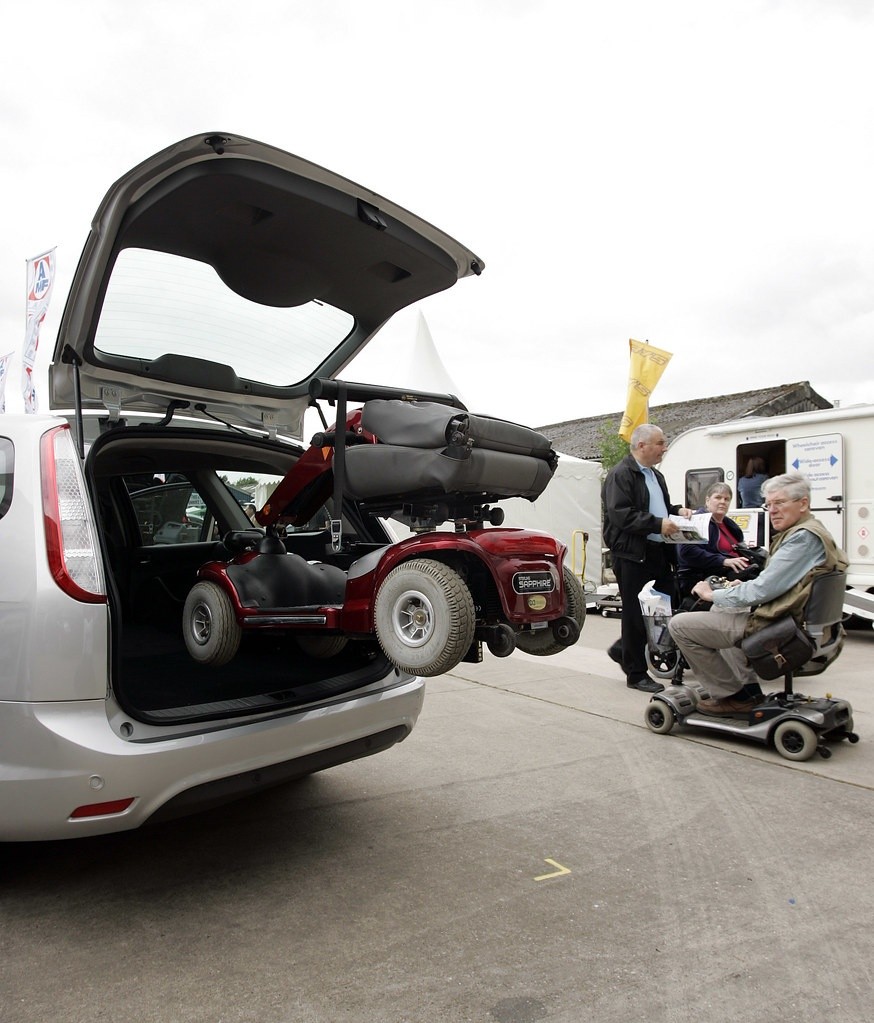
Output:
[761, 497, 801, 511]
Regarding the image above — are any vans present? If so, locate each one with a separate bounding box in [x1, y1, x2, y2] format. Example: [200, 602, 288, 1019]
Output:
[0, 129, 488, 847]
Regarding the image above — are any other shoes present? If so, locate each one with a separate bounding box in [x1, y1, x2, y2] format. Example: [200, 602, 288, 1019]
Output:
[607, 648, 625, 675]
[754, 693, 766, 703]
[696, 693, 759, 720]
[627, 674, 665, 693]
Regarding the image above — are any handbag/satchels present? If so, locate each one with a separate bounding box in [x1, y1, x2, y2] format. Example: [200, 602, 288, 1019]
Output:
[740, 614, 814, 681]
[732, 541, 769, 567]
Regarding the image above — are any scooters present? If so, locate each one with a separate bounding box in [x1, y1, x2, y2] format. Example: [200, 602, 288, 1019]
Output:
[643, 546, 771, 680]
[179, 377, 588, 671]
[644, 578, 861, 761]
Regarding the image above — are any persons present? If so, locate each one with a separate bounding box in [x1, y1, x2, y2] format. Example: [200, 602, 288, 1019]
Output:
[600, 424, 693, 693]
[737, 458, 771, 509]
[668, 472, 849, 722]
[675, 482, 758, 603]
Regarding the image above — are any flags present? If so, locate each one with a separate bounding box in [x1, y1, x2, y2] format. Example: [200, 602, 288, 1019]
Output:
[617, 338, 675, 445]
[0, 352, 14, 415]
[20, 249, 58, 413]
